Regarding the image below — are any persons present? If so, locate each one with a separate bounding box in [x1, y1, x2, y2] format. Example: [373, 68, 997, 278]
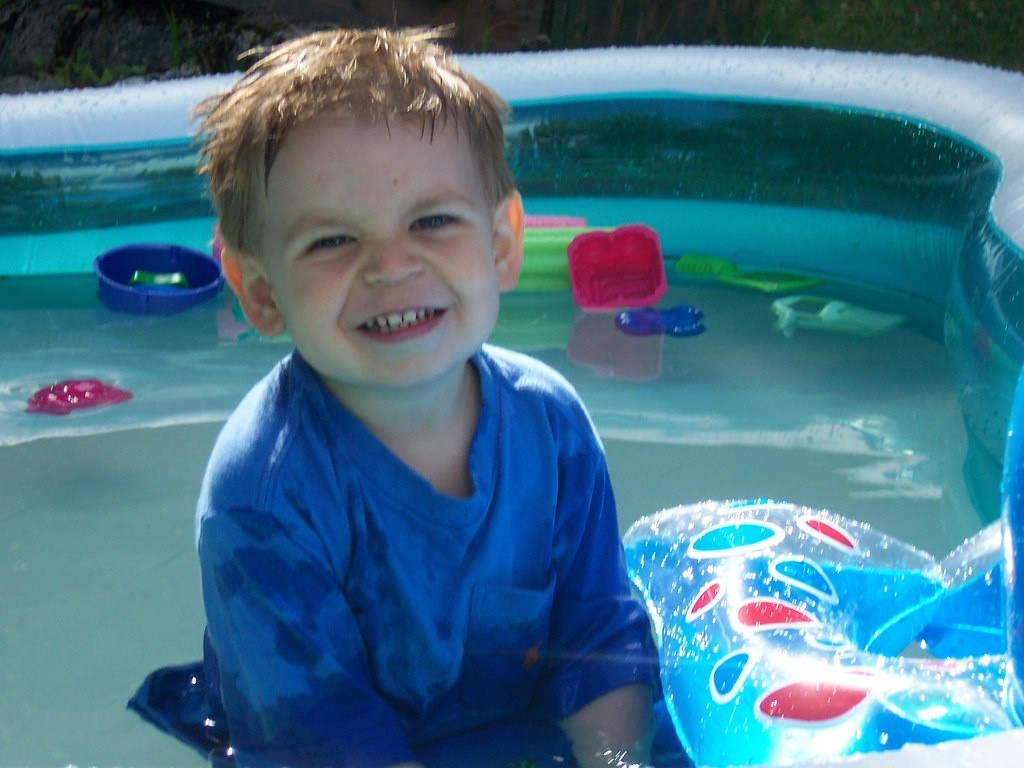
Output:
[124, 27, 661, 767]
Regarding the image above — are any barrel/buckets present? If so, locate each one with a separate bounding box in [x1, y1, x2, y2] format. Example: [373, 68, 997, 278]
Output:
[93, 241, 225, 317]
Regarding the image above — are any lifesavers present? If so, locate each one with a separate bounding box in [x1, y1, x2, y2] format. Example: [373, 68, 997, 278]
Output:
[620, 362, 1024, 768]
